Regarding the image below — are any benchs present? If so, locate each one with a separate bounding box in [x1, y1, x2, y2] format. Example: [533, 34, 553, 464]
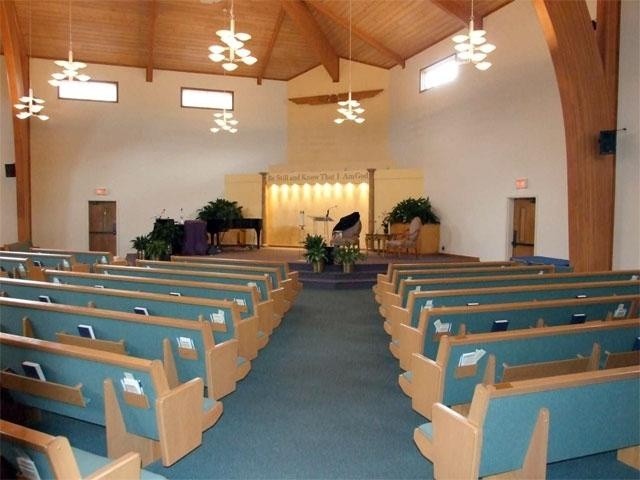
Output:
[373, 250, 639, 479]
[0, 419, 166, 480]
[0, 240, 304, 467]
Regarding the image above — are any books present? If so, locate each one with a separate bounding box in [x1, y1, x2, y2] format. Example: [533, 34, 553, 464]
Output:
[77, 325, 96, 339]
[467, 303, 479, 306]
[632, 337, 640, 351]
[576, 295, 587, 298]
[491, 320, 509, 332]
[133, 307, 149, 315]
[572, 314, 586, 324]
[34, 260, 41, 267]
[169, 292, 181, 296]
[21, 360, 46, 383]
[38, 295, 51, 302]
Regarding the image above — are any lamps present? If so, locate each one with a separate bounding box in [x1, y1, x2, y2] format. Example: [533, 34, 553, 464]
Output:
[47, 0, 90, 86]
[451, 0, 496, 71]
[208, 1, 258, 71]
[210, 71, 240, 134]
[13, 2, 50, 121]
[333, 0, 366, 125]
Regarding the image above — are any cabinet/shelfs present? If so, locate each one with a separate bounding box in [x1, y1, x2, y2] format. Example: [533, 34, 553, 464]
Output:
[390, 222, 440, 256]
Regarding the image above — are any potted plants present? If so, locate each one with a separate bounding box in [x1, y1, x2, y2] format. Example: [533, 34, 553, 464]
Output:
[335, 240, 368, 274]
[298, 233, 328, 273]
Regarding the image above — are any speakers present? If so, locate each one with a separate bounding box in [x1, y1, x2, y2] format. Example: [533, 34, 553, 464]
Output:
[5, 162, 16, 179]
[598, 130, 615, 156]
